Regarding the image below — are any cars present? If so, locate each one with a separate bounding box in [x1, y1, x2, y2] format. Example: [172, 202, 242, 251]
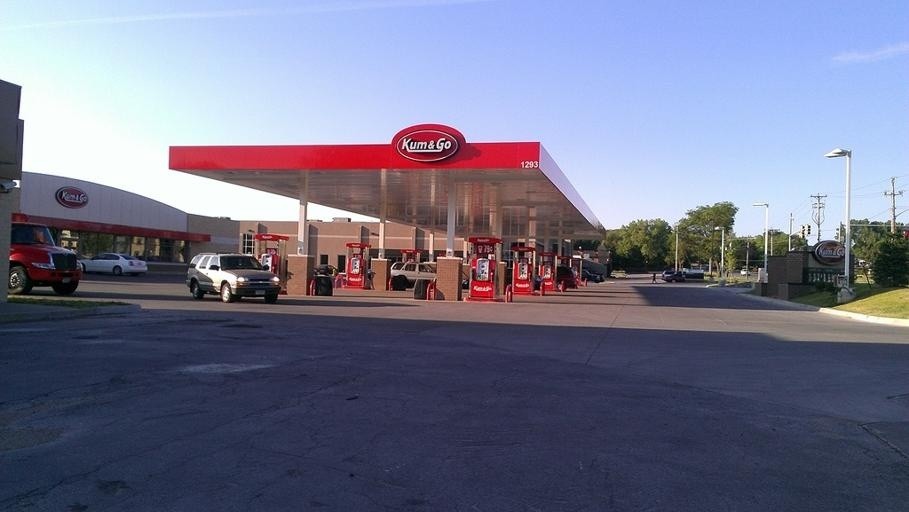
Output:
[662, 269, 686, 282]
[462, 261, 577, 290]
[741, 269, 750, 276]
[582, 269, 604, 283]
[80, 252, 148, 275]
[316, 263, 338, 274]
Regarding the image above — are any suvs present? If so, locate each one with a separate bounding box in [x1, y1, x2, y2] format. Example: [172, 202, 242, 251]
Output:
[186, 251, 281, 303]
[8, 221, 83, 295]
[390, 262, 436, 287]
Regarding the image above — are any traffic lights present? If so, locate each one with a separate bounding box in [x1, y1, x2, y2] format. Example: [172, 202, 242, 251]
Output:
[808, 225, 810, 233]
[801, 225, 804, 236]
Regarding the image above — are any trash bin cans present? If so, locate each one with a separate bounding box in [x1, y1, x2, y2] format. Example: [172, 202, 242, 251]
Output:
[315, 276, 333, 297]
[392, 276, 407, 291]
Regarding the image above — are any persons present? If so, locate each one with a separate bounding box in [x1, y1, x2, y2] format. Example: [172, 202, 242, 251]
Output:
[652, 273, 656, 284]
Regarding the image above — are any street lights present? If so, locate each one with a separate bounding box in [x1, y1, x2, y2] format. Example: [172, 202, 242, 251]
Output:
[752, 203, 768, 271]
[825, 147, 851, 286]
[713, 225, 725, 273]
